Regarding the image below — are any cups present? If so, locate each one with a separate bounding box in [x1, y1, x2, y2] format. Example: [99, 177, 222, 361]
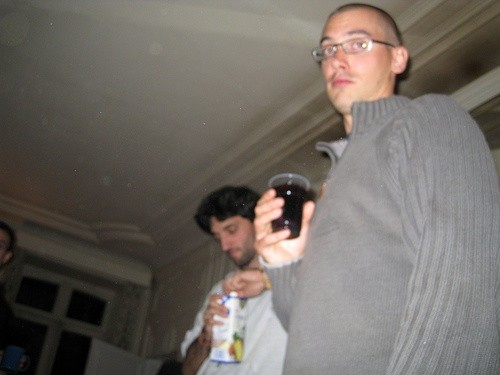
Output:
[268, 173, 311, 239]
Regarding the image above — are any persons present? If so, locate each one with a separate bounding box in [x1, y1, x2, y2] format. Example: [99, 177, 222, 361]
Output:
[169, 184, 288, 374]
[0, 224, 27, 375]
[254, 2, 500, 375]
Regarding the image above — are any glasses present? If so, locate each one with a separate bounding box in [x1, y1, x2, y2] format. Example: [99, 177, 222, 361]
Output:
[310, 38, 396, 61]
